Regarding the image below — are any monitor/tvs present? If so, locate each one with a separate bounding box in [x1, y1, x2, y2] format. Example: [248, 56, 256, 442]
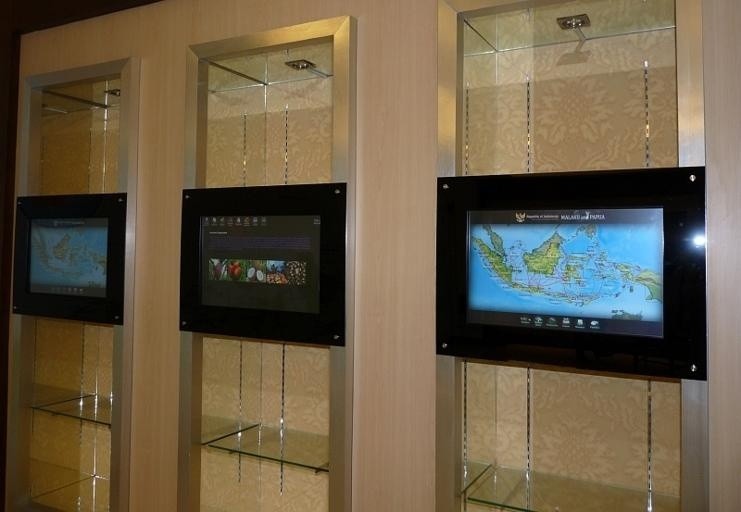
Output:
[179, 180, 348, 350]
[10, 191, 128, 327]
[434, 165, 707, 383]
